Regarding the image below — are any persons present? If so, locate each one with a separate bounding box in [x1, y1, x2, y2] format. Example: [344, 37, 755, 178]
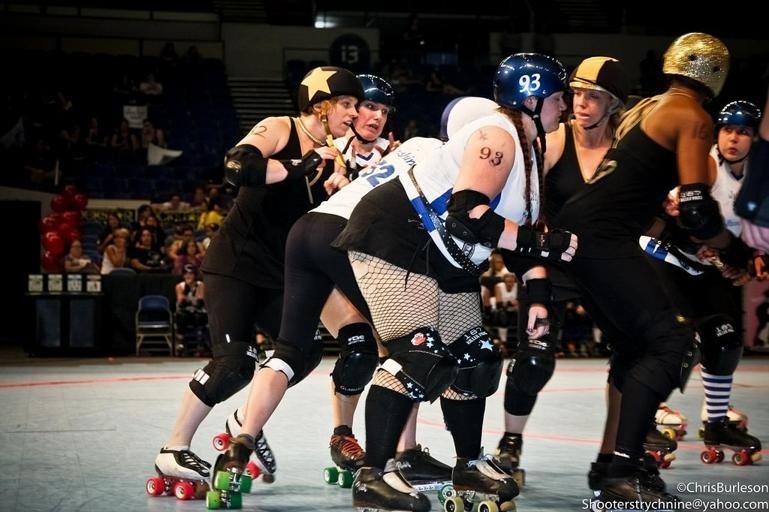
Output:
[639, 94, 762, 457]
[328, 50, 581, 511]
[492, 55, 638, 492]
[319, 62, 402, 471]
[209, 94, 506, 494]
[653, 398, 752, 429]
[1, 44, 769, 356]
[153, 62, 353, 481]
[549, 29, 758, 509]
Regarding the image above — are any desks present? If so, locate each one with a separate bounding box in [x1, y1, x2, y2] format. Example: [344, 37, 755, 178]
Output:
[23, 291, 108, 358]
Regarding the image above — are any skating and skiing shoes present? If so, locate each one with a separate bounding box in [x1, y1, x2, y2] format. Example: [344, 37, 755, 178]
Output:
[698, 399, 762, 465]
[589, 402, 689, 511]
[323, 435, 524, 512]
[146, 410, 277, 510]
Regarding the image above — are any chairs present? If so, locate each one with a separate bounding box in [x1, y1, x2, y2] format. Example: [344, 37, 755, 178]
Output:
[134, 294, 174, 356]
[82, 223, 210, 275]
[480, 275, 521, 349]
[318, 324, 342, 352]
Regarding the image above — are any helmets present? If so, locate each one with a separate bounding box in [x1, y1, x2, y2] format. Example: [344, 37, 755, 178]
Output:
[660, 32, 730, 103]
[356, 74, 397, 116]
[716, 101, 763, 128]
[494, 51, 568, 111]
[298, 66, 364, 112]
[568, 55, 628, 108]
[440, 96, 501, 141]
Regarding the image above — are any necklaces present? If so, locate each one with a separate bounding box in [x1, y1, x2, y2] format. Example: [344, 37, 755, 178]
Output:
[295, 114, 333, 147]
